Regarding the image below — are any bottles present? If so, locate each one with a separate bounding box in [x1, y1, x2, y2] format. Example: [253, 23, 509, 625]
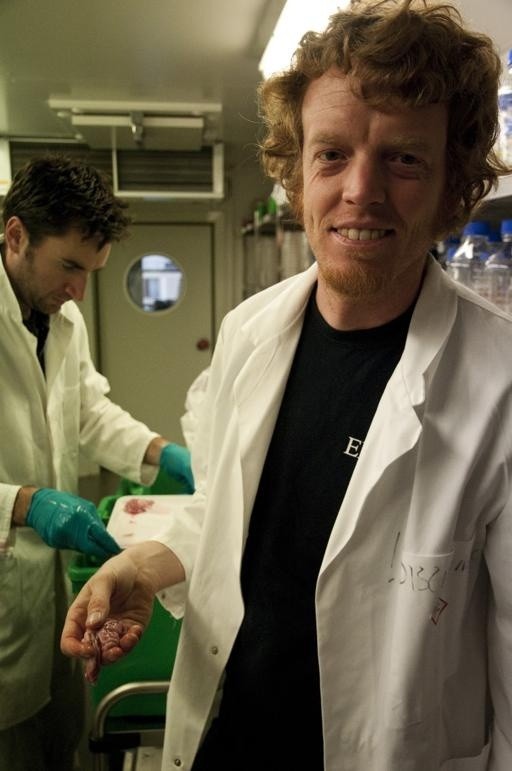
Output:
[497, 49, 512, 148]
[439, 219, 512, 309]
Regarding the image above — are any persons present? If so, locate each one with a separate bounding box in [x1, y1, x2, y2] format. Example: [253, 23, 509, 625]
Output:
[1, 149, 197, 771]
[57, 1, 512, 771]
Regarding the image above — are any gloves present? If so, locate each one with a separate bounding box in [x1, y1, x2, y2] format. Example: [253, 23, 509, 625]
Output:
[159, 441, 195, 491]
[25, 486, 120, 558]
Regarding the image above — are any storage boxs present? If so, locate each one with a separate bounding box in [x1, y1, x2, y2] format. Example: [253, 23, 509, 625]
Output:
[64, 494, 182, 720]
